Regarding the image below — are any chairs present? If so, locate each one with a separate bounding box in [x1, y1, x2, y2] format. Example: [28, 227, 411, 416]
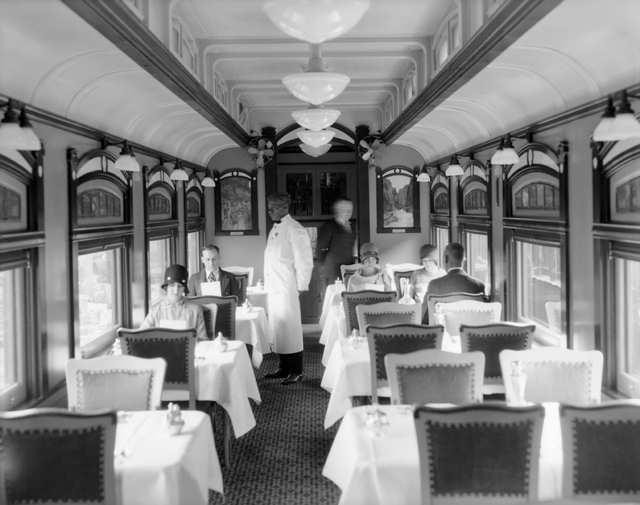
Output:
[1, 264, 278, 505]
[315, 261, 640, 505]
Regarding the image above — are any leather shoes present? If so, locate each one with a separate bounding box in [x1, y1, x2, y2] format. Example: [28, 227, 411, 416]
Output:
[265, 365, 292, 380]
[279, 373, 304, 385]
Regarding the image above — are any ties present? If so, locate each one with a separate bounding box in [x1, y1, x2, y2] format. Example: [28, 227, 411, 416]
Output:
[207, 272, 216, 283]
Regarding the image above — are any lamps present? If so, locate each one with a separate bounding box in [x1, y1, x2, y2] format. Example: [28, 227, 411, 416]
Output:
[416, 161, 441, 183]
[193, 168, 222, 190]
[299, 143, 332, 158]
[0, 97, 43, 155]
[291, 107, 341, 132]
[490, 132, 535, 167]
[444, 151, 475, 177]
[592, 88, 640, 143]
[258, 2, 372, 46]
[296, 129, 337, 145]
[158, 154, 190, 182]
[282, 43, 351, 105]
[103, 136, 140, 173]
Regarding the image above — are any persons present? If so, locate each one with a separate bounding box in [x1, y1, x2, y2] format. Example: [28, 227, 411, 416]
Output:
[262, 191, 314, 387]
[137, 263, 209, 340]
[187, 243, 244, 307]
[315, 195, 357, 305]
[347, 242, 393, 291]
[408, 242, 449, 304]
[420, 243, 486, 323]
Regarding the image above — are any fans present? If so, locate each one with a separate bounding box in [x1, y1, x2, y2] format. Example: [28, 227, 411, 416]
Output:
[356, 132, 389, 167]
[243, 127, 277, 169]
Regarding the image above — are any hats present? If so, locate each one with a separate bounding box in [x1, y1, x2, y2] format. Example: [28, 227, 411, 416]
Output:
[419, 243, 439, 265]
[359, 242, 380, 263]
[160, 264, 190, 294]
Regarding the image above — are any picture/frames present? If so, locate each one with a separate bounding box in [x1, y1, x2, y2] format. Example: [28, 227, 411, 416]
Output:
[373, 164, 422, 234]
[211, 167, 261, 238]
